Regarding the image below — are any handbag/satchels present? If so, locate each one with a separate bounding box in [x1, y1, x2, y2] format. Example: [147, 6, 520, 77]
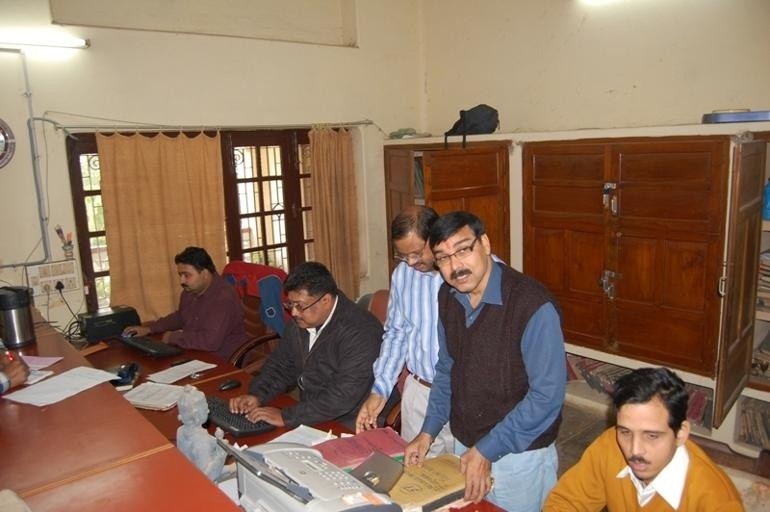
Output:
[445, 104, 498, 135]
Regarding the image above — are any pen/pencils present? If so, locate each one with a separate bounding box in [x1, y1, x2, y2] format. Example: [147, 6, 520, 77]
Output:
[5, 351, 14, 363]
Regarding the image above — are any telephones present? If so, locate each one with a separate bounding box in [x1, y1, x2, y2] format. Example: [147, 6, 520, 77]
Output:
[105, 363, 138, 384]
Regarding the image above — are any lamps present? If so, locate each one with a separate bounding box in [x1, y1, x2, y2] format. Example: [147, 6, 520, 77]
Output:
[0, 30, 89, 50]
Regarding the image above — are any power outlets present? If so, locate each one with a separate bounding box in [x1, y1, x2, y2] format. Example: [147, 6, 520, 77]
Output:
[40, 280, 51, 293]
[65, 278, 76, 292]
[53, 279, 64, 293]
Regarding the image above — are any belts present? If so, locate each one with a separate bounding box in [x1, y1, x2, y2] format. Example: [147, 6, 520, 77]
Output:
[410, 373, 432, 388]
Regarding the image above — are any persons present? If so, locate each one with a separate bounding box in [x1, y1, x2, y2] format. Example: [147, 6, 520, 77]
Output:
[542, 366, 746, 512]
[229, 261, 401, 428]
[121, 246, 251, 368]
[355, 203, 507, 461]
[0, 350, 30, 392]
[402, 211, 568, 512]
[176, 383, 228, 485]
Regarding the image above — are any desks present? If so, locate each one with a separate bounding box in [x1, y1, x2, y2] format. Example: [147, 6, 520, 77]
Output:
[0, 303, 174, 502]
[69, 321, 243, 395]
[123, 368, 507, 511]
[20, 446, 243, 512]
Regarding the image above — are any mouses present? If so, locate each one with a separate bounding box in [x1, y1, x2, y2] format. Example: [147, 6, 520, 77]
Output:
[217, 380, 241, 391]
[123, 331, 137, 338]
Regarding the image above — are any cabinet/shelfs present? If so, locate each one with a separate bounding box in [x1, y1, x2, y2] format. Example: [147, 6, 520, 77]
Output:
[517, 131, 734, 381]
[384, 139, 510, 290]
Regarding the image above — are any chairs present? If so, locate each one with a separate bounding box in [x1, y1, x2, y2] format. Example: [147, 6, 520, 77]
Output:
[353, 289, 402, 434]
[219, 259, 290, 367]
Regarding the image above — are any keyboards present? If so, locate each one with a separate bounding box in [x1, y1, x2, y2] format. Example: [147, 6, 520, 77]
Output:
[207, 395, 275, 438]
[121, 332, 177, 357]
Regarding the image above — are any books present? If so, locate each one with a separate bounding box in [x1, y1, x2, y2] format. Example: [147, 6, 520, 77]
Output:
[752, 251, 770, 376]
[313, 424, 495, 512]
[123, 381, 184, 412]
[568, 354, 712, 431]
[739, 398, 769, 451]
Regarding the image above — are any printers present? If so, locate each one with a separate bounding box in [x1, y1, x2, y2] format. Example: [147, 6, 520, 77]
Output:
[76, 303, 141, 344]
[212, 437, 408, 512]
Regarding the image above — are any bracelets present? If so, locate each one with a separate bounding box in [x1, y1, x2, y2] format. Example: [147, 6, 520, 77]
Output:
[0, 370, 10, 395]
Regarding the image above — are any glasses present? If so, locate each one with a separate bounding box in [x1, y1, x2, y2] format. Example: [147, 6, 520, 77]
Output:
[282, 292, 329, 313]
[393, 238, 427, 262]
[436, 238, 478, 264]
